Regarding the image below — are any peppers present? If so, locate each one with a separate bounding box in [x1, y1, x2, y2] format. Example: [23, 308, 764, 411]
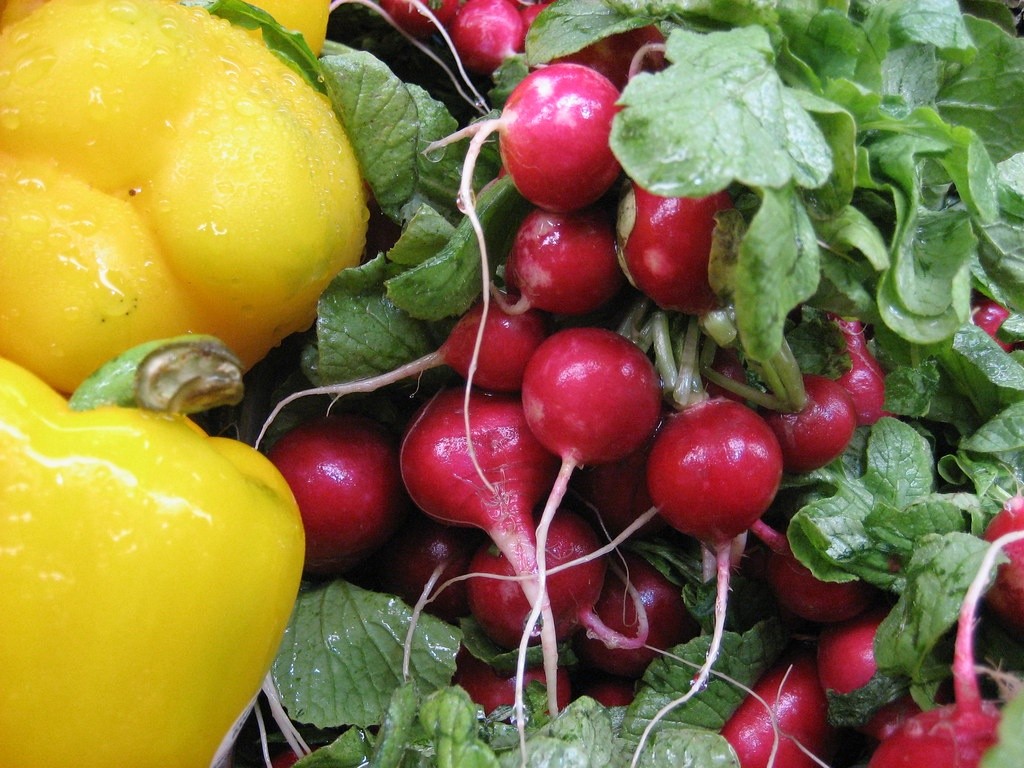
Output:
[0, 0, 372, 768]
